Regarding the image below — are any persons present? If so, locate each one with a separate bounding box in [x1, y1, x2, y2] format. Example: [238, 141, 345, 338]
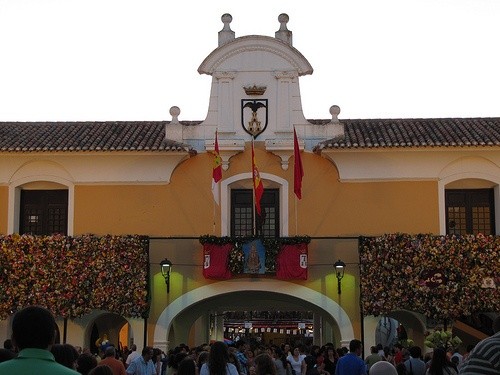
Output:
[246, 246, 262, 273]
[0, 306, 500, 375]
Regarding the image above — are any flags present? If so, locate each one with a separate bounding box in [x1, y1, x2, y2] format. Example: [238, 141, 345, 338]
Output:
[293, 130, 304, 201]
[251, 142, 264, 214]
[213, 131, 222, 206]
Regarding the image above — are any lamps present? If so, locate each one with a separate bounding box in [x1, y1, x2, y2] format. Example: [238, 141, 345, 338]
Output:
[159, 258, 172, 293]
[333, 259, 346, 294]
